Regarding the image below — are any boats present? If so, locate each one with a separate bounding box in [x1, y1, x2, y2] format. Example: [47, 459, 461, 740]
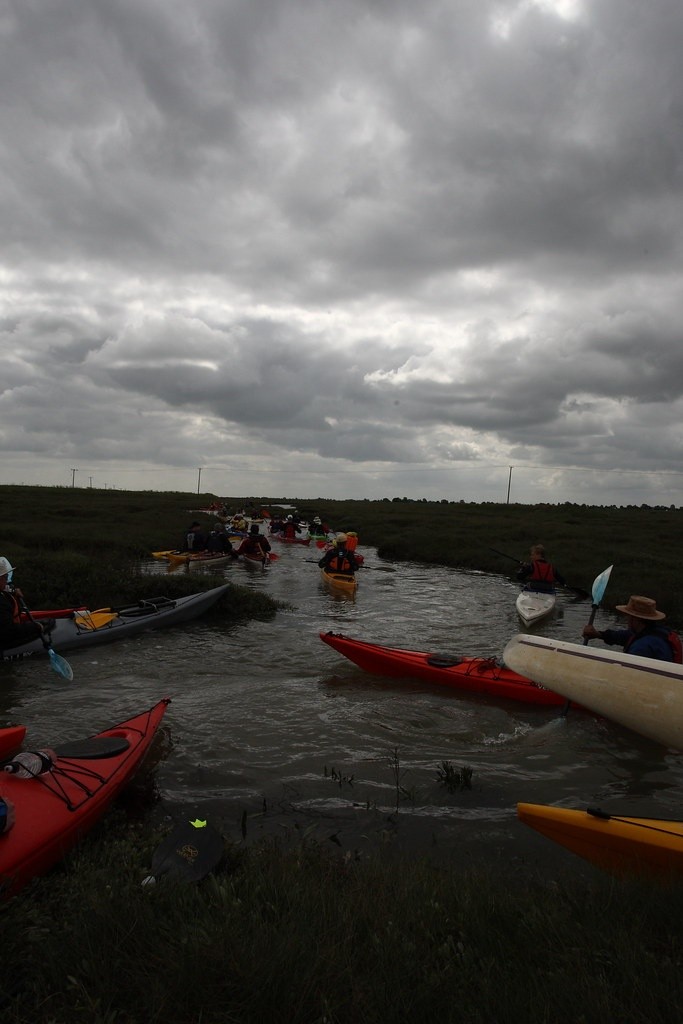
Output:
[320, 566, 365, 599]
[218, 514, 336, 551]
[320, 628, 589, 714]
[501, 634, 683, 753]
[228, 535, 278, 571]
[153, 546, 235, 573]
[0, 719, 32, 763]
[18, 605, 90, 623]
[514, 802, 683, 893]
[515, 587, 559, 628]
[0, 583, 235, 671]
[0, 696, 173, 909]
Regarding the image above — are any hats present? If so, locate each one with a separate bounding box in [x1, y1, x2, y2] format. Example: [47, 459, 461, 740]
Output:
[287, 515, 293, 519]
[616, 596, 665, 621]
[0, 557, 16, 577]
[214, 523, 223, 529]
[335, 532, 347, 542]
[189, 522, 200, 529]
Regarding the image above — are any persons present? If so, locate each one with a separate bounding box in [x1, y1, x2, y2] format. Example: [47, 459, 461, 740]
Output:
[318, 533, 359, 573]
[234, 525, 271, 555]
[583, 595, 674, 662]
[270, 514, 325, 538]
[219, 506, 263, 531]
[0, 563, 43, 649]
[517, 544, 555, 593]
[181, 522, 232, 552]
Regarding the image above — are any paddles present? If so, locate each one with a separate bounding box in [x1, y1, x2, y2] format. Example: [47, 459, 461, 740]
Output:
[75, 596, 177, 630]
[582, 565, 615, 648]
[299, 560, 396, 573]
[261, 508, 299, 539]
[489, 546, 588, 599]
[7, 569, 74, 685]
